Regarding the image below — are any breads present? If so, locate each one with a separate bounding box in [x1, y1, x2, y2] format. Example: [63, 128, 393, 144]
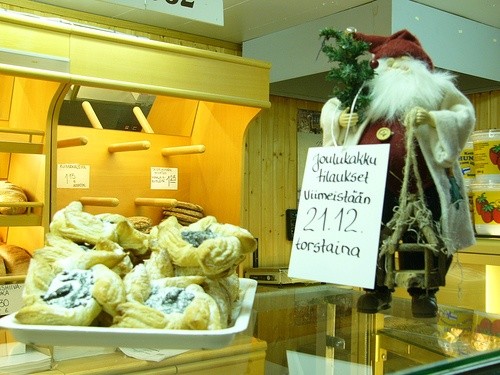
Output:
[0, 176, 258, 330]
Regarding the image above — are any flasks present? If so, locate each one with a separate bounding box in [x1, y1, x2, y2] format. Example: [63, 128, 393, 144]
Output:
[471, 129, 500, 184]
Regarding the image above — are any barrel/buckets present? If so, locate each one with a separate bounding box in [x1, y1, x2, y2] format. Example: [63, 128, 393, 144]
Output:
[472, 186, 500, 236]
[457, 142, 475, 185]
[466, 187, 476, 235]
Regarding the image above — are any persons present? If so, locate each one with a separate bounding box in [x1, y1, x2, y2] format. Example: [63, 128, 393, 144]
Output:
[320, 24, 476, 319]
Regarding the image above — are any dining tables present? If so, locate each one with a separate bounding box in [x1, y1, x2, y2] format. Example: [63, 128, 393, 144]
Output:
[0, 282, 500, 375]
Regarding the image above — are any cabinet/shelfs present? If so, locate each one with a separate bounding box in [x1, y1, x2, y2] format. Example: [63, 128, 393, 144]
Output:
[1, 19, 273, 315]
